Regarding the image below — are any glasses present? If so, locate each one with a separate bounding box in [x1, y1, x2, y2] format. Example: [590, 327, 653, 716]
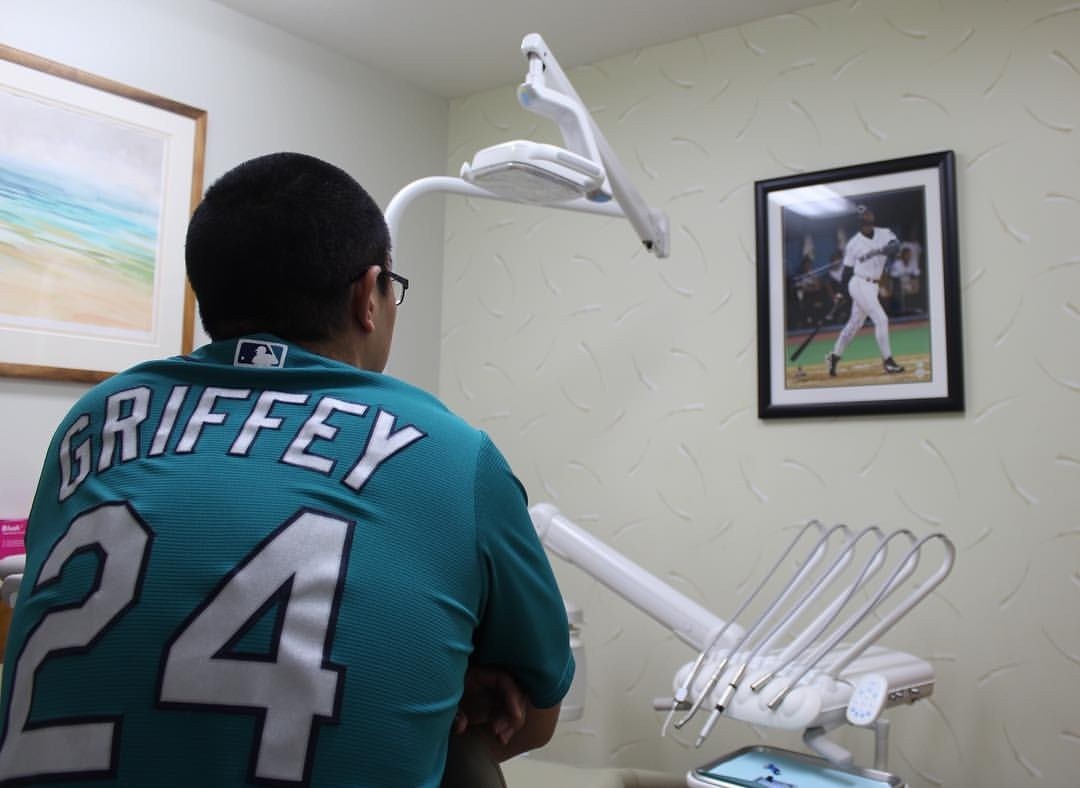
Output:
[350, 270, 409, 305]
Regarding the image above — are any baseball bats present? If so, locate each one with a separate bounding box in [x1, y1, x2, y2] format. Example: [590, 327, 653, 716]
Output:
[789, 307, 833, 362]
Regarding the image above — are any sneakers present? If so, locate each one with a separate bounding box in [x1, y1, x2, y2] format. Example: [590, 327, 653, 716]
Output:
[884, 358, 904, 373]
[827, 355, 838, 376]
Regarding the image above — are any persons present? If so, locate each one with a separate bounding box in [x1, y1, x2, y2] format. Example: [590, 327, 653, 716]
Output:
[796, 199, 925, 377]
[0, 153, 577, 788]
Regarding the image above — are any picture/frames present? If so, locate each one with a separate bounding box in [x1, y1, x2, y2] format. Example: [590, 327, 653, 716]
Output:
[755, 148, 966, 419]
[0, 42, 208, 384]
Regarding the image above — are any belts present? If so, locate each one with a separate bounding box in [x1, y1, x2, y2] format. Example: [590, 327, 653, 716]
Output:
[859, 276, 880, 284]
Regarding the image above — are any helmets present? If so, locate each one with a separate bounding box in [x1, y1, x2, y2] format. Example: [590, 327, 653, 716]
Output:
[856, 205, 876, 225]
